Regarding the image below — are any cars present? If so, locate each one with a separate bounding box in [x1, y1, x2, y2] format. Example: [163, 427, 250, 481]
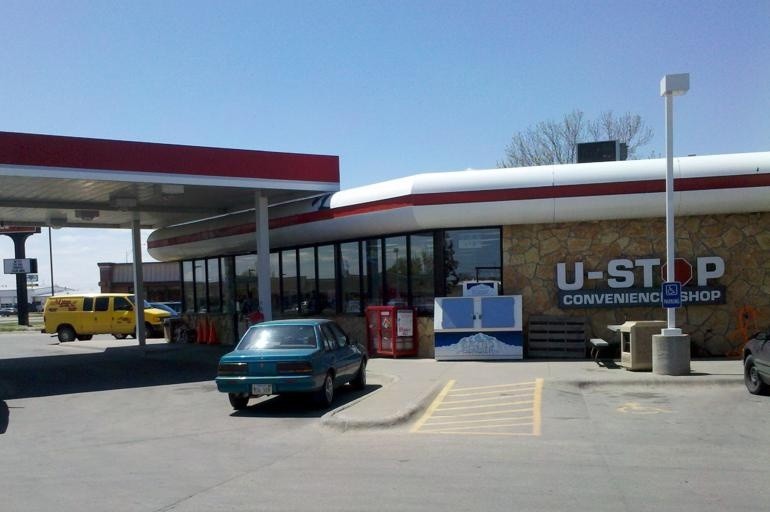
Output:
[741, 324, 770, 394]
[0, 308, 18, 317]
[148, 300, 182, 316]
[215, 318, 368, 412]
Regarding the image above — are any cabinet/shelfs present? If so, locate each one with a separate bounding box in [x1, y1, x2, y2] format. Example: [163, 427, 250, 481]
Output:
[365, 305, 418, 358]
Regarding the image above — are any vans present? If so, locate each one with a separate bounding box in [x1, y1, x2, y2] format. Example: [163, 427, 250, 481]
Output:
[41, 291, 171, 344]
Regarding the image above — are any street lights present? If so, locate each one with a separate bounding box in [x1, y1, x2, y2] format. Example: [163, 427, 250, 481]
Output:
[659, 71, 689, 335]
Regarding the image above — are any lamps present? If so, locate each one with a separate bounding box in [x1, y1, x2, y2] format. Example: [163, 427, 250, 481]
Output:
[154, 184, 186, 202]
[108, 197, 137, 211]
[75, 210, 99, 221]
[46, 219, 67, 230]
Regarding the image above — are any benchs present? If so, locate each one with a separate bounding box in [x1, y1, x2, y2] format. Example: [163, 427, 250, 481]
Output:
[589, 338, 618, 367]
